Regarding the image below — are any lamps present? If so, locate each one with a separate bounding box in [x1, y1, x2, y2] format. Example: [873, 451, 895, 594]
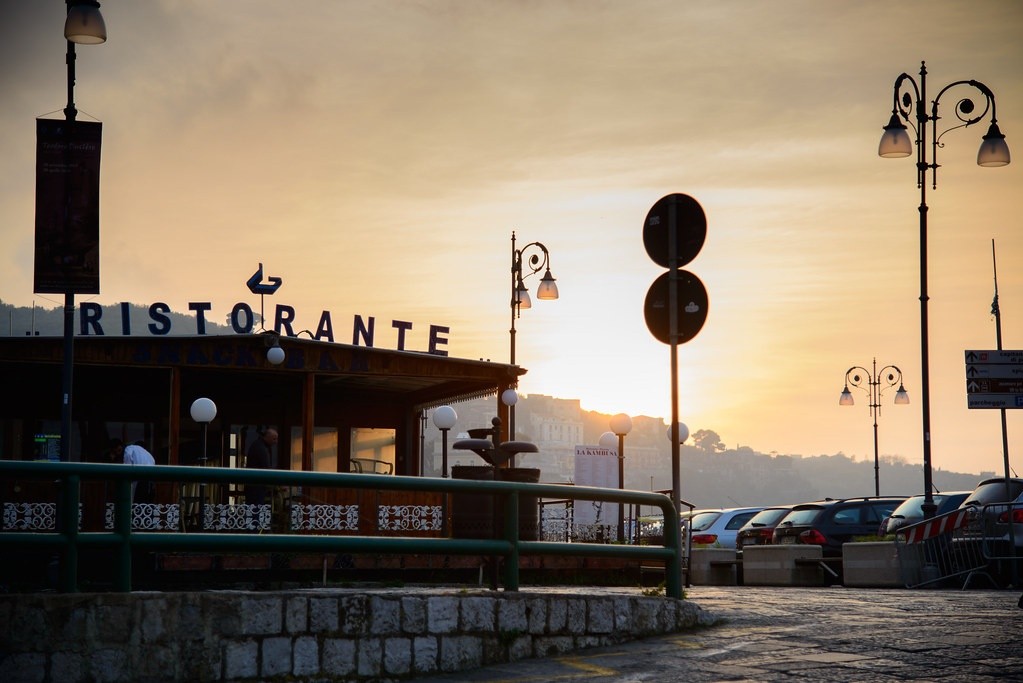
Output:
[262, 330, 285, 365]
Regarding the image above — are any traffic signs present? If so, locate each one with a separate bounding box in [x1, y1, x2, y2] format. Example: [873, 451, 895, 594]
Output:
[963, 349, 1023, 410]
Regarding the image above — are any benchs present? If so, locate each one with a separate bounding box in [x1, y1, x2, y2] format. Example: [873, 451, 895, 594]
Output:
[710, 559, 743, 566]
[794, 557, 842, 564]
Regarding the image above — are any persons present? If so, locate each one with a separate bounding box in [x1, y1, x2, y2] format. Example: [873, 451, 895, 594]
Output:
[246, 427, 289, 504]
[106, 439, 155, 503]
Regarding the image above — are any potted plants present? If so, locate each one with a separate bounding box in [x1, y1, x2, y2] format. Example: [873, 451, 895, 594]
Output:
[742, 537, 824, 586]
[841, 534, 923, 587]
[682, 543, 736, 585]
[632, 526, 666, 567]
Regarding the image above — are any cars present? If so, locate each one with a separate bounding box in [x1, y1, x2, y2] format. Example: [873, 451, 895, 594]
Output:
[641, 474, 1023, 589]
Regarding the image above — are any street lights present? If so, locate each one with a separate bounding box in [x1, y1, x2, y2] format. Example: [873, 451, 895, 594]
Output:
[876, 58, 1012, 474]
[837, 356, 910, 495]
[62, 0, 110, 464]
[190, 398, 217, 532]
[510, 229, 559, 467]
[609, 413, 632, 546]
[433, 406, 457, 587]
